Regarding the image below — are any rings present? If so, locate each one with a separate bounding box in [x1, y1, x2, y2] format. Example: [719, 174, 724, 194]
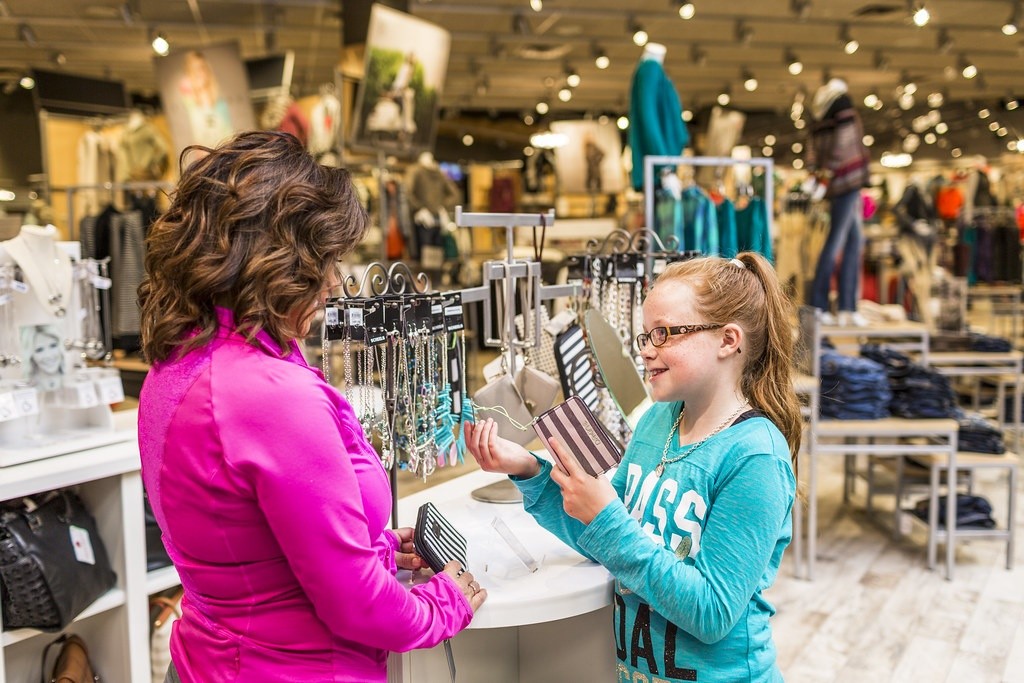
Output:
[469, 584, 476, 595]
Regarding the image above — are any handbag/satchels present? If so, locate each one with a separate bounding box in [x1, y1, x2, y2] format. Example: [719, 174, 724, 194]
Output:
[149, 585, 185, 683]
[1, 490, 118, 634]
[144, 487, 175, 571]
[41, 635, 101, 683]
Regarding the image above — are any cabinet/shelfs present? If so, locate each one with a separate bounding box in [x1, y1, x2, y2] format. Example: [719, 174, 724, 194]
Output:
[0, 384, 386, 683]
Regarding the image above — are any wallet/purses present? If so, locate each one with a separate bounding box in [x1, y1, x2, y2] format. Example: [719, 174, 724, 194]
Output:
[470, 304, 600, 445]
[412, 502, 468, 578]
[533, 397, 622, 481]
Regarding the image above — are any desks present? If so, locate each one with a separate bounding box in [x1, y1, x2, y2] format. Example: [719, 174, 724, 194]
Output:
[783, 276, 1024, 583]
[397, 449, 615, 683]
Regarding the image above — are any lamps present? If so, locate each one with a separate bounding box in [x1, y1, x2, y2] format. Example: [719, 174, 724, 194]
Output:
[535, 0, 1024, 120]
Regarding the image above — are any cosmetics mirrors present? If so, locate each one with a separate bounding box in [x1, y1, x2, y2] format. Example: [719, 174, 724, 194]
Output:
[584, 308, 652, 434]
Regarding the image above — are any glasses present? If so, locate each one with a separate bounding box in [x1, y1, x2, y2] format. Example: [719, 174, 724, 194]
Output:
[636, 324, 743, 351]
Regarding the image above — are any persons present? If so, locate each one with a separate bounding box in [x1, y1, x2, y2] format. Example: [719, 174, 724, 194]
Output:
[394, 52, 416, 150]
[585, 132, 604, 191]
[25, 325, 68, 378]
[181, 50, 220, 143]
[465, 252, 802, 683]
[137, 132, 488, 683]
[806, 79, 990, 326]
[408, 156, 463, 285]
[631, 43, 688, 252]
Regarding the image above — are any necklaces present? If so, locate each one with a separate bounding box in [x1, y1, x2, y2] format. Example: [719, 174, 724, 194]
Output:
[591, 257, 649, 448]
[17, 232, 68, 317]
[323, 323, 475, 482]
[656, 397, 749, 477]
